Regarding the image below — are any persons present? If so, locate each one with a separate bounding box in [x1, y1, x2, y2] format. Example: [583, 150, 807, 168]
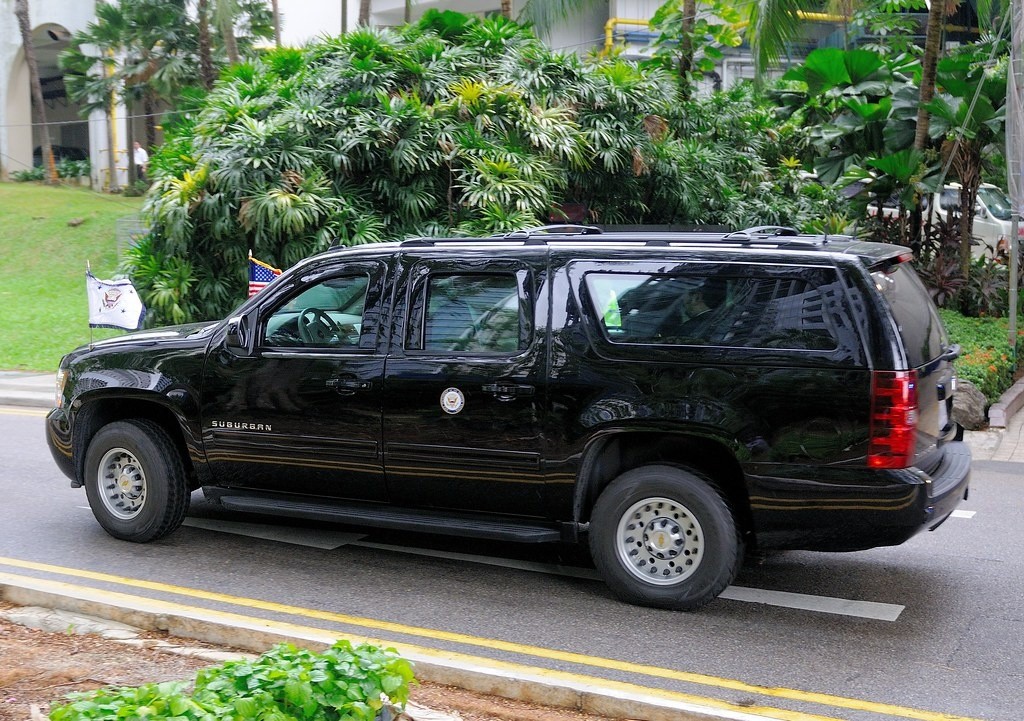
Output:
[676, 277, 726, 343]
[336, 322, 362, 345]
[132, 141, 149, 183]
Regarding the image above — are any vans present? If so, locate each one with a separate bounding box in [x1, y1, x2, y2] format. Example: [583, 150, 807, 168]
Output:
[868, 182, 1024, 268]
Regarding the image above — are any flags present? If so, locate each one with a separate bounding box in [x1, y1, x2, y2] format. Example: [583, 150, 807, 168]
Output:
[86, 271, 146, 331]
[248, 257, 282, 298]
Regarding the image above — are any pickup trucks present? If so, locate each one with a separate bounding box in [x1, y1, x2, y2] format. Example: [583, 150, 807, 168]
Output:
[45, 226, 973, 613]
